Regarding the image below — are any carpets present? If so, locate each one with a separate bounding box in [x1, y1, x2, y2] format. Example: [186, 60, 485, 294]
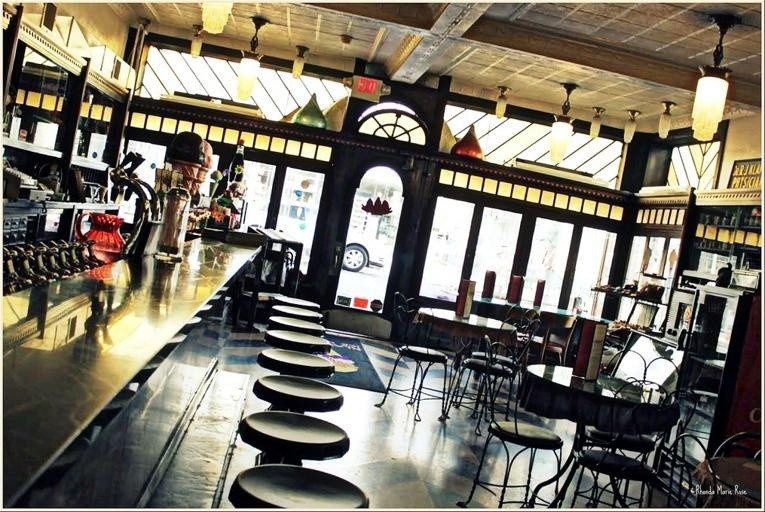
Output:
[325, 334, 388, 394]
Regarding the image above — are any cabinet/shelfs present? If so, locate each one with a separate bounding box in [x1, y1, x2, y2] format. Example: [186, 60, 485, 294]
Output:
[4, 1, 135, 249]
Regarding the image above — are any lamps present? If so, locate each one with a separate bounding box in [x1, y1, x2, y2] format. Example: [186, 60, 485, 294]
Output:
[187, 0, 311, 102]
[494, 13, 744, 167]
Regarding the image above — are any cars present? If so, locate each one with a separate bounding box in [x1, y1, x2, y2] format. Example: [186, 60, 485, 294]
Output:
[341, 229, 386, 272]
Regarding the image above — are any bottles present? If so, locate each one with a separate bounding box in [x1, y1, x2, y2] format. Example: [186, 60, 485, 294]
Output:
[227, 139, 245, 191]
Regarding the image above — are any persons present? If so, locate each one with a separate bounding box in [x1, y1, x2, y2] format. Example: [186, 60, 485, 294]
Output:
[190, 181, 201, 208]
[205, 180, 242, 230]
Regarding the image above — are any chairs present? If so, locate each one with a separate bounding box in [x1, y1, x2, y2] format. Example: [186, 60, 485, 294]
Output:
[376, 288, 716, 506]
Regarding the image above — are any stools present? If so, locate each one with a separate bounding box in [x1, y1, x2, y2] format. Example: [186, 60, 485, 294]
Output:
[226, 293, 371, 511]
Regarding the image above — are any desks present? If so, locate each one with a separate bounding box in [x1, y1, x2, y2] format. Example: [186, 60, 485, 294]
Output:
[695, 453, 761, 509]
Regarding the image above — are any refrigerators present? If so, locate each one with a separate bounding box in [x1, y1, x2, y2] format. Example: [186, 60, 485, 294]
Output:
[648, 284, 761, 508]
